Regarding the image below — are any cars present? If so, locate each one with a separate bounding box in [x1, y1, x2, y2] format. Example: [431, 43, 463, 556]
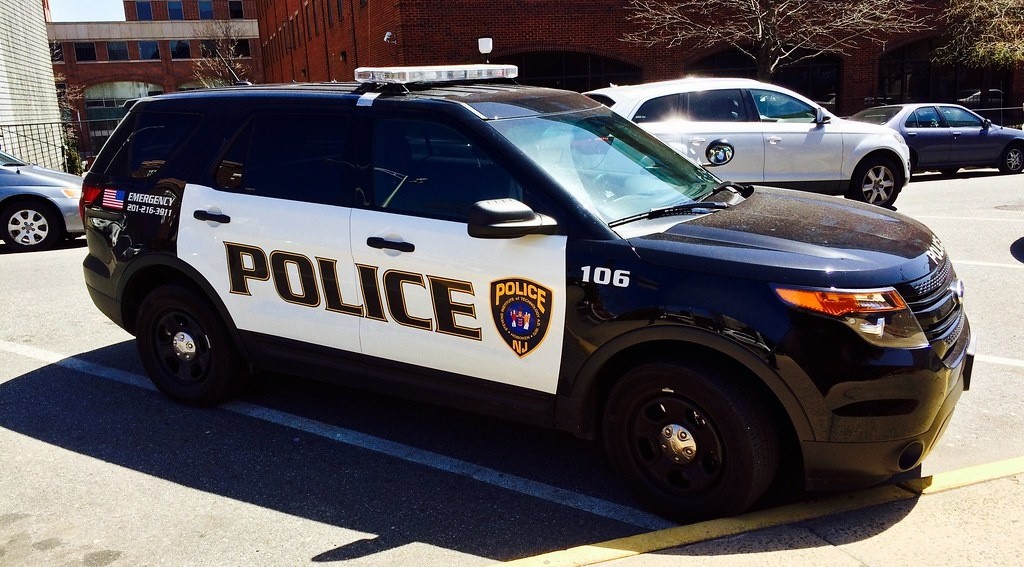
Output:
[849, 102, 1024, 175]
[0, 150, 85, 251]
[77, 64, 974, 523]
[535, 77, 911, 205]
[812, 93, 837, 112]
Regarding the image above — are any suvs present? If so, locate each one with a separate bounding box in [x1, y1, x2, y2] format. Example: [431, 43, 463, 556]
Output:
[955, 89, 1007, 107]
